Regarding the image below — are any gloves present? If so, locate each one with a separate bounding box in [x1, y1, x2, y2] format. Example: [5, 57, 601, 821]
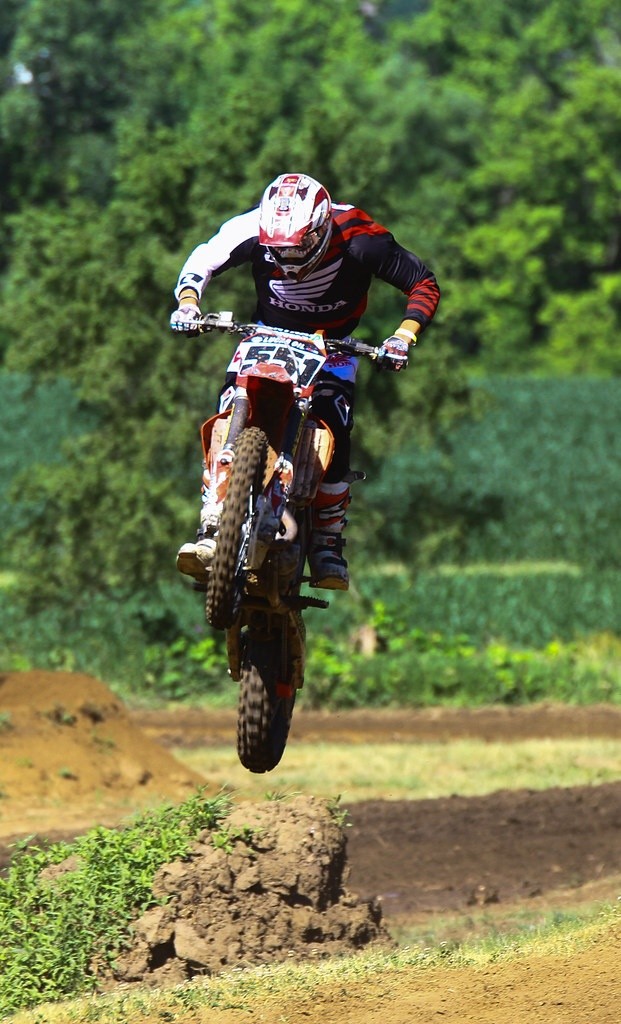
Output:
[169, 304, 203, 338]
[377, 336, 409, 372]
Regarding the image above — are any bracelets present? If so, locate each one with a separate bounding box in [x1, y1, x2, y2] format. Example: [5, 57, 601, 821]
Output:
[395, 328, 417, 346]
[179, 295, 199, 305]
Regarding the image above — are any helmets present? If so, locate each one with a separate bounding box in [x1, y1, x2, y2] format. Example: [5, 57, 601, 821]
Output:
[259, 173, 331, 284]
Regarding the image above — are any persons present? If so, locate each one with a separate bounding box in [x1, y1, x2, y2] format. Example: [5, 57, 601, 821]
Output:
[170, 173, 441, 591]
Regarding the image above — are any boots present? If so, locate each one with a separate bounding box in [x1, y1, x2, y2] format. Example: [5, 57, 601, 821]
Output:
[308, 470, 366, 591]
[176, 448, 235, 582]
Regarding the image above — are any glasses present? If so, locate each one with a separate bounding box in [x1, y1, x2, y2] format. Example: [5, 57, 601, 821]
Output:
[268, 185, 332, 265]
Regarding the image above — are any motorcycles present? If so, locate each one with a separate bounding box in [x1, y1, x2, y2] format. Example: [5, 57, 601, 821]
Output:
[168, 312, 412, 774]
